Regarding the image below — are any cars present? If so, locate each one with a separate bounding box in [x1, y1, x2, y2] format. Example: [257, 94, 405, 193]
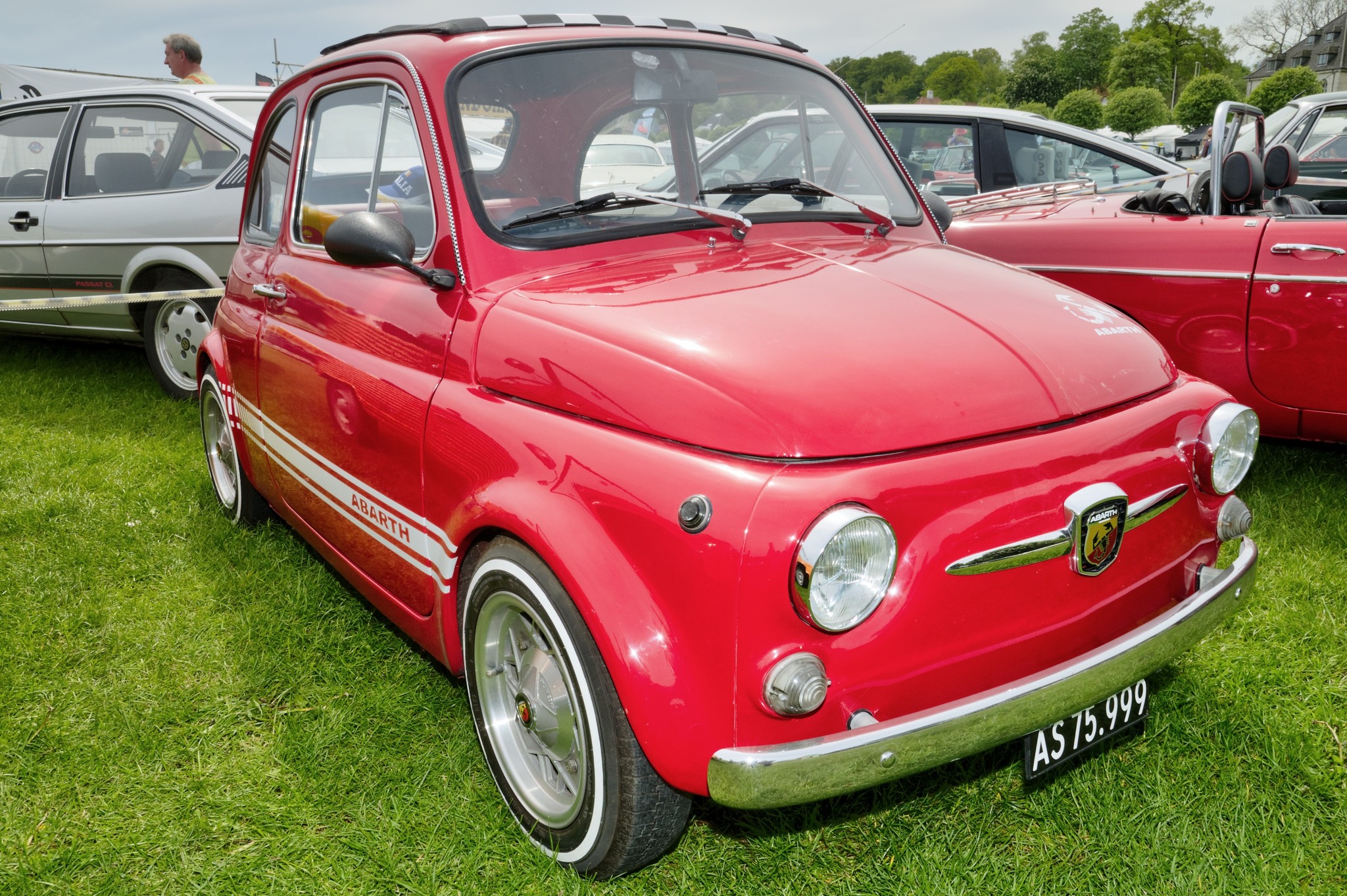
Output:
[2, 82, 539, 401]
[1184, 82, 1347, 196]
[681, 102, 1180, 224]
[194, 7, 1265, 864]
[559, 133, 677, 202]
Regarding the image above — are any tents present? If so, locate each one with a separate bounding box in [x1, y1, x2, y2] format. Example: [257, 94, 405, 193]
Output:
[693, 113, 737, 134]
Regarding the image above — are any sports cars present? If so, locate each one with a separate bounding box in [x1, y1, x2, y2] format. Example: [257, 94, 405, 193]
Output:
[925, 98, 1345, 450]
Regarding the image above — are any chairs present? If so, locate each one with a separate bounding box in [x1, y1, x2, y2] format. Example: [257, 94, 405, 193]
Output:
[90, 150, 149, 198]
[1261, 141, 1324, 217]
[1217, 148, 1265, 220]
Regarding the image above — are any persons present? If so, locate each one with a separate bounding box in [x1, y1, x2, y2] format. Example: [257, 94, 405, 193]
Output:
[1191, 135, 1210, 159]
[1202, 127, 1213, 159]
[161, 30, 237, 170]
[365, 165, 432, 206]
[147, 137, 167, 177]
[947, 126, 974, 147]
[490, 114, 514, 150]
[1330, 147, 1346, 159]
